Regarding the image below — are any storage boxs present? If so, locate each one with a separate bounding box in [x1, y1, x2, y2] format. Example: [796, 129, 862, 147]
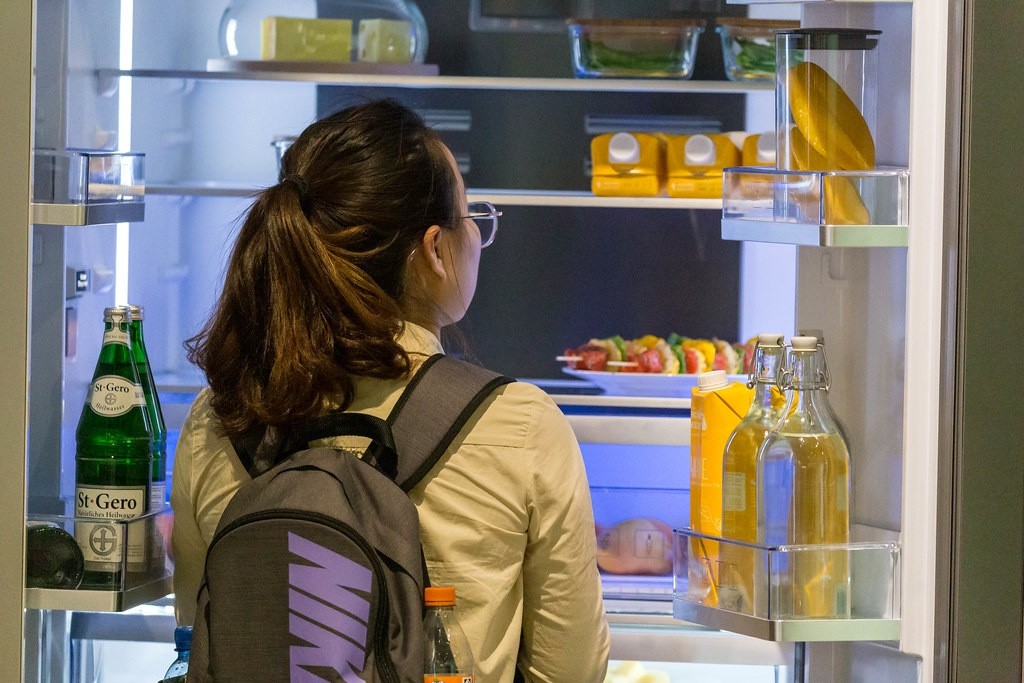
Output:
[714, 16, 803, 81]
[566, 17, 706, 77]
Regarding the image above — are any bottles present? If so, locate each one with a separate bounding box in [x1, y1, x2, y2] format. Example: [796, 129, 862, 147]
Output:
[162, 625, 194, 683]
[716, 329, 853, 619]
[775, 29, 883, 225]
[419, 585, 477, 682]
[25, 303, 169, 592]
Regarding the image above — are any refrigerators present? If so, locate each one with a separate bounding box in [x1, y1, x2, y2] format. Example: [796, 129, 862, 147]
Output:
[0, 2, 1024, 683]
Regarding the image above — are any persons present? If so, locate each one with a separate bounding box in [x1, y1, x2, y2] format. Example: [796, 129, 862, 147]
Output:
[170, 99, 612, 683]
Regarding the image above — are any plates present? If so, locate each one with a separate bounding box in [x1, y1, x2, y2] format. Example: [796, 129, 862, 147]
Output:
[561, 363, 749, 398]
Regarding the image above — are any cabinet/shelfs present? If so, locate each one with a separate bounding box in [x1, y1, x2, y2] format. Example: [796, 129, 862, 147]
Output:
[96, 64, 777, 597]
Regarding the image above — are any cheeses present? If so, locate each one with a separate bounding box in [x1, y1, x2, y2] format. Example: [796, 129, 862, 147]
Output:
[260, 15, 354, 60]
[356, 18, 414, 62]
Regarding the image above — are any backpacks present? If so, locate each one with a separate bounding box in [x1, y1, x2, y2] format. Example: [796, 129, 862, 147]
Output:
[185, 353, 517, 683]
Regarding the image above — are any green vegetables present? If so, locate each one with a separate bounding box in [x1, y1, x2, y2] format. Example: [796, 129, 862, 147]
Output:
[728, 37, 812, 71]
[569, 38, 690, 78]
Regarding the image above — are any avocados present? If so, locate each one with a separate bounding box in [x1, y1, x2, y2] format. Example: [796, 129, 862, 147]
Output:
[26, 526, 85, 589]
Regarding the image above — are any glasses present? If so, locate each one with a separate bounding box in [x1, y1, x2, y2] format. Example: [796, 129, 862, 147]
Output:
[418, 200, 503, 249]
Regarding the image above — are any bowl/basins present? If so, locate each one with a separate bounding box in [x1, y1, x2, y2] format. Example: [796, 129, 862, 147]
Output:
[569, 24, 706, 79]
[716, 26, 790, 82]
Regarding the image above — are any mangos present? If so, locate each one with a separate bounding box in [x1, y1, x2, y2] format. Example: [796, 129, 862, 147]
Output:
[593, 517, 679, 575]
